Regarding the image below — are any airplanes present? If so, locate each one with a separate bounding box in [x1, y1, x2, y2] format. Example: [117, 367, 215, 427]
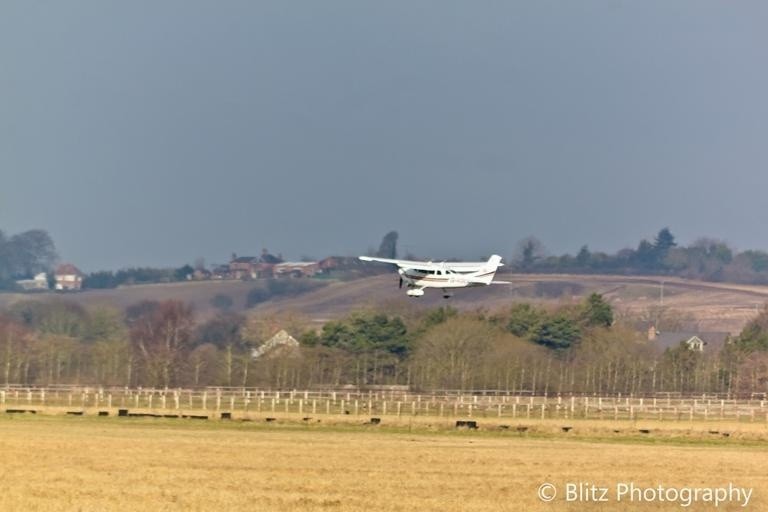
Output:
[354, 249, 513, 299]
